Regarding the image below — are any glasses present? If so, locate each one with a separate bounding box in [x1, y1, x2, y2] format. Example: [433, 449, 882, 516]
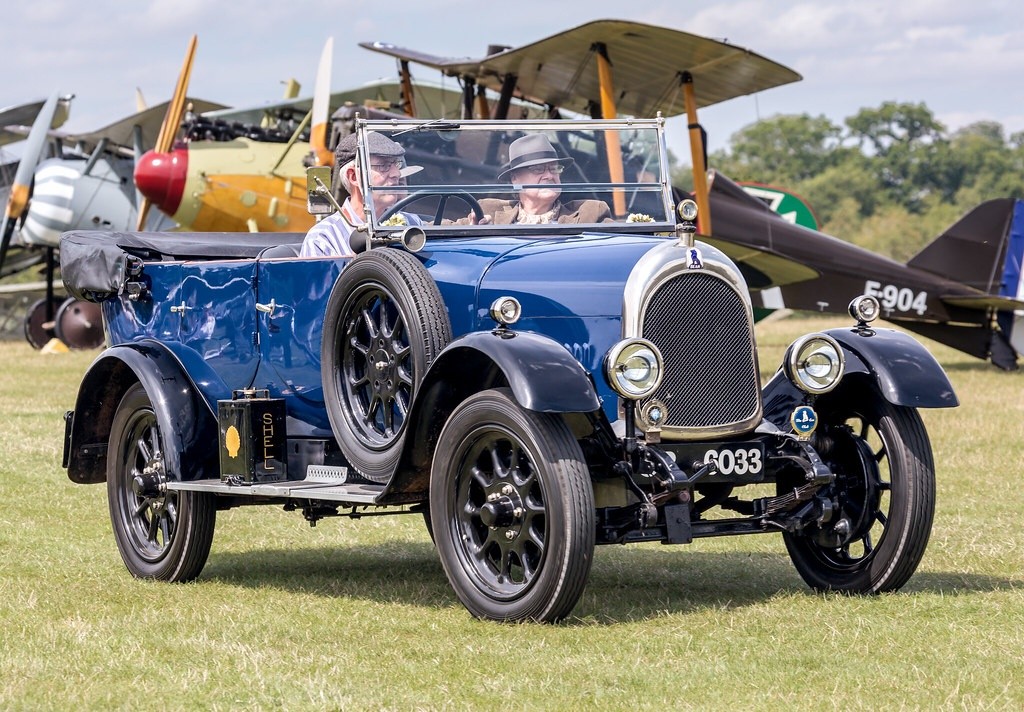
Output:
[371, 159, 404, 172]
[526, 163, 566, 175]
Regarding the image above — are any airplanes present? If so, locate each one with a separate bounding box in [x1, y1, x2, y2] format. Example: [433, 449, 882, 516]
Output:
[1, 16, 1023, 373]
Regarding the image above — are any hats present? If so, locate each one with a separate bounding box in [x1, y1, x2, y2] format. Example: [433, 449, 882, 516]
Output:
[394, 142, 424, 178]
[335, 130, 406, 171]
[497, 133, 575, 181]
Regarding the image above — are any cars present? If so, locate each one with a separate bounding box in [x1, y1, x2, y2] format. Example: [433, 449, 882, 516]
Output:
[57, 106, 961, 626]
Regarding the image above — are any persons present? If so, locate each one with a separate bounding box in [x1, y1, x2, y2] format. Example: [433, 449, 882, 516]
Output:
[298, 133, 422, 258]
[421, 133, 620, 225]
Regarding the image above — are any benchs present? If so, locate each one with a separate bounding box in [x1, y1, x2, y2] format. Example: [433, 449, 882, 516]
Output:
[255, 243, 302, 258]
